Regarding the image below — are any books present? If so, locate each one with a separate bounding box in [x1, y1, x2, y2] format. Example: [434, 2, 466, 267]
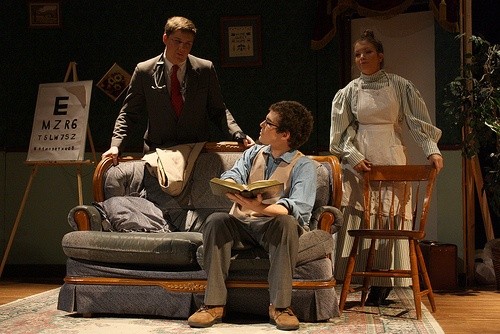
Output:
[208, 178, 284, 203]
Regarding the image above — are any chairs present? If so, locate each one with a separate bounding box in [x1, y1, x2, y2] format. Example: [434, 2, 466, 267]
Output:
[339, 165, 437, 320]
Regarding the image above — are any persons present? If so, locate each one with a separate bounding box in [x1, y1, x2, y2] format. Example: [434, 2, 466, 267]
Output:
[328, 28, 443, 307]
[187, 101, 316, 330]
[101, 16, 257, 168]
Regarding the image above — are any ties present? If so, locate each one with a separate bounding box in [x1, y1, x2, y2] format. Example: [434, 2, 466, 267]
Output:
[169, 65, 184, 119]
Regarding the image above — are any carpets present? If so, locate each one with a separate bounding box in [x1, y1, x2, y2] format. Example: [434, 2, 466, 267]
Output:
[0, 287, 445, 334]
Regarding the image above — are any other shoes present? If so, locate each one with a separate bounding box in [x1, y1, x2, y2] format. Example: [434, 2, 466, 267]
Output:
[365, 283, 393, 306]
[269, 292, 299, 330]
[188, 305, 223, 327]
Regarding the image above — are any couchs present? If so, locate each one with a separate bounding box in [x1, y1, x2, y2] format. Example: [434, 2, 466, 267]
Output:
[57, 141, 341, 323]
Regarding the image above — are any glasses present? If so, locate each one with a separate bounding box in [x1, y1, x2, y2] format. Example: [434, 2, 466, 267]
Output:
[264, 117, 283, 130]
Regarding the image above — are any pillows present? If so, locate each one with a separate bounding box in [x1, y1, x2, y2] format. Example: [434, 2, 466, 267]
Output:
[92, 192, 178, 232]
